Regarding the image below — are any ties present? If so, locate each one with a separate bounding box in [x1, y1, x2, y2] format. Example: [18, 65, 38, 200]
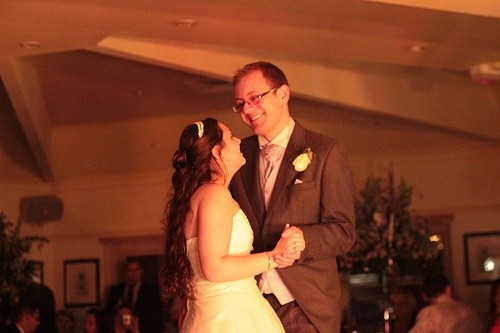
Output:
[126, 287, 134, 308]
[263, 144, 282, 183]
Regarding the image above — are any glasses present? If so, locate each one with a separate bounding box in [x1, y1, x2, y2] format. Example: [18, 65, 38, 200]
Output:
[232, 84, 280, 114]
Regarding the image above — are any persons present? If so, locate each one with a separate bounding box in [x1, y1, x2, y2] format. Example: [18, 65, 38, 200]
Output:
[0, 260, 74, 333]
[489, 279, 500, 333]
[164, 117, 306, 333]
[228, 61, 356, 333]
[84, 259, 163, 333]
[407, 273, 487, 333]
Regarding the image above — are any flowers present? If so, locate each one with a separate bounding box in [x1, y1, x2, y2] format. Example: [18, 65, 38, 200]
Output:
[293, 145, 315, 173]
[339, 165, 440, 278]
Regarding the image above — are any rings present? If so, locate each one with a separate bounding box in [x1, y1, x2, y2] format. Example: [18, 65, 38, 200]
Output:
[295, 247, 297, 252]
[294, 241, 296, 248]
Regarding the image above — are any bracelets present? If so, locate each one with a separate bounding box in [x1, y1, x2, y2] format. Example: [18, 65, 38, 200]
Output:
[266, 252, 271, 271]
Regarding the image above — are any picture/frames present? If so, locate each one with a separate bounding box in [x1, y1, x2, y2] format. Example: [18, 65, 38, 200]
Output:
[64, 257, 103, 307]
[464, 229, 500, 286]
